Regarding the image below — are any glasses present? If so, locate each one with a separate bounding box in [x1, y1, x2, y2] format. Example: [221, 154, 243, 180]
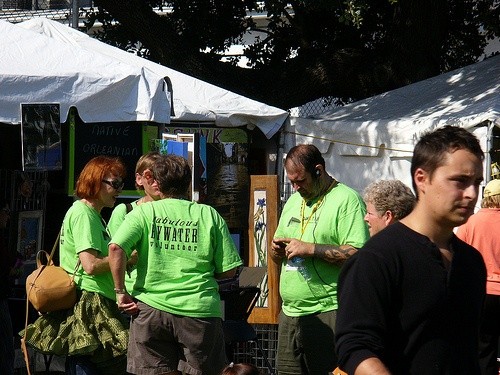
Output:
[103, 177, 125, 190]
[139, 169, 156, 179]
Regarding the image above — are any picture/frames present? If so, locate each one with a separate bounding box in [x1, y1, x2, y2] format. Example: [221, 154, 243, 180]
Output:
[246, 175, 281, 324]
[17, 211, 44, 264]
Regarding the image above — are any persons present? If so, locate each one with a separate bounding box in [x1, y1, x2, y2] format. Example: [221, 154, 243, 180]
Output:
[221, 362, 262, 375]
[364, 179, 415, 237]
[103, 153, 167, 375]
[459, 179, 500, 370]
[18, 153, 137, 375]
[334, 125, 487, 375]
[268, 144, 370, 375]
[109, 152, 243, 375]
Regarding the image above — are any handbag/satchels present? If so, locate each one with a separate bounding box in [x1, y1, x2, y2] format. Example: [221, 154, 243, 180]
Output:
[25, 249, 82, 314]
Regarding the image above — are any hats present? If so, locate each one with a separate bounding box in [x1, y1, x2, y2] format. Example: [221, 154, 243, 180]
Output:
[484, 179, 500, 197]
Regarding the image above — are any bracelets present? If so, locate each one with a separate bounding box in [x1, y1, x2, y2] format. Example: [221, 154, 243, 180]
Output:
[114, 287, 128, 293]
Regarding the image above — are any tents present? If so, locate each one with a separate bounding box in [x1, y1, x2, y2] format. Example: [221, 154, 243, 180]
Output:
[0, 16, 288, 137]
[280, 55, 500, 231]
